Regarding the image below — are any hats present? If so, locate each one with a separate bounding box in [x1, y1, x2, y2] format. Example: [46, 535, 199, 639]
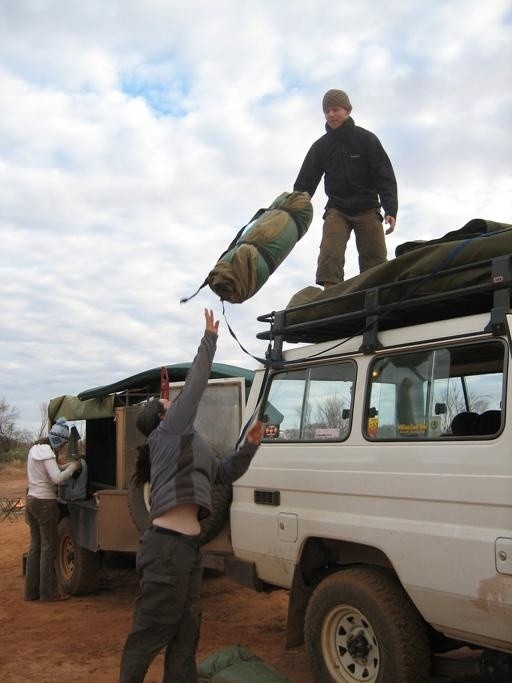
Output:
[48, 417, 70, 448]
[323, 89, 351, 114]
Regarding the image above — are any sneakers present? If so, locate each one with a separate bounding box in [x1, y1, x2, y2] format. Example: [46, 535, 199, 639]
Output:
[53, 591, 70, 600]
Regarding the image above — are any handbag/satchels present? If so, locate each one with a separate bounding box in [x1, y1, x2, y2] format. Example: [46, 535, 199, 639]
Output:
[60, 455, 88, 500]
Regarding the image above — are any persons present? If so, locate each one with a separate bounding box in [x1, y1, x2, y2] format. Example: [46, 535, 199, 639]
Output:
[119, 304, 265, 683]
[294, 87, 397, 284]
[23, 415, 81, 603]
